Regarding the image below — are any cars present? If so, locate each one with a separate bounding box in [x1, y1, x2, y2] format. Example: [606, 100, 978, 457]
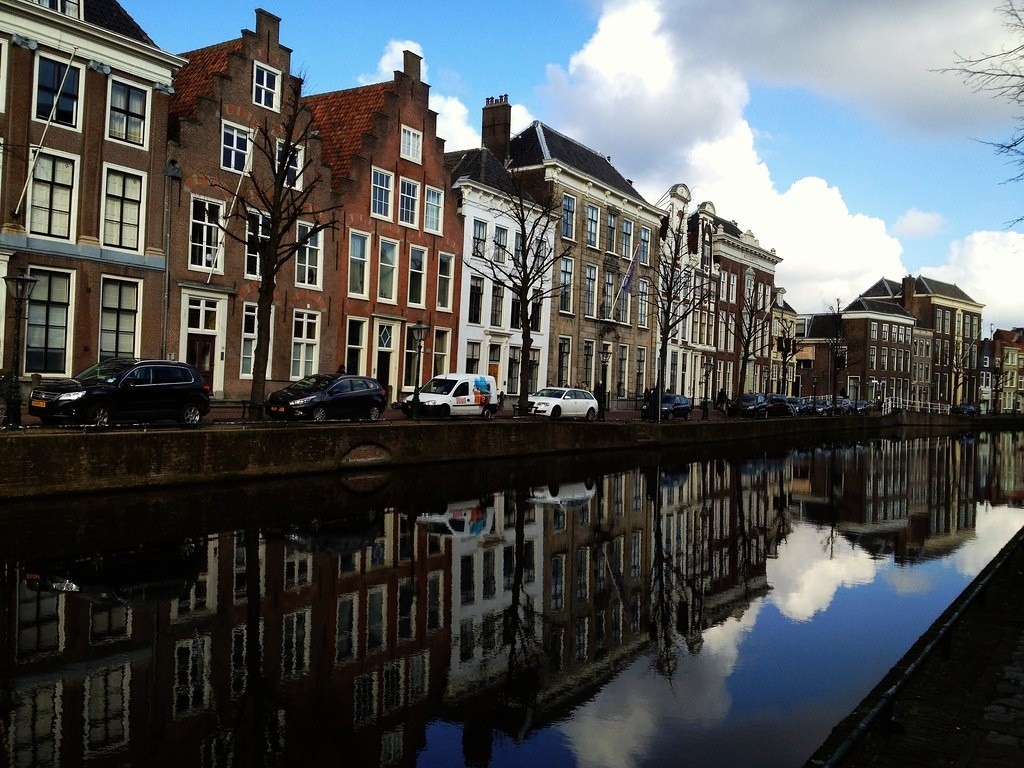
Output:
[266, 513, 384, 551]
[727, 391, 872, 419]
[263, 371, 388, 424]
[641, 391, 691, 422]
[515, 386, 599, 423]
[521, 477, 596, 508]
[950, 403, 976, 418]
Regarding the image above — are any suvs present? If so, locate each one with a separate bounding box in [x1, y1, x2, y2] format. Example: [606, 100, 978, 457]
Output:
[20, 531, 207, 608]
[28, 357, 212, 430]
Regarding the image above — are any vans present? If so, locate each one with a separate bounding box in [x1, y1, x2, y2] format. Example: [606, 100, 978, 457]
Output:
[400, 372, 499, 420]
[399, 497, 496, 539]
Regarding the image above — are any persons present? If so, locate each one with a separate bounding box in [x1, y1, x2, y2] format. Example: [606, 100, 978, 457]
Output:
[716, 388, 727, 415]
[336, 364, 346, 375]
[574, 382, 589, 391]
[644, 387, 671, 404]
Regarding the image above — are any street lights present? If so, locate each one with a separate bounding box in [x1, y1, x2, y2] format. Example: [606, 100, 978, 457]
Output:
[2, 271, 40, 430]
[812, 374, 819, 416]
[408, 319, 430, 420]
[700, 360, 714, 420]
[763, 367, 771, 397]
[853, 379, 861, 416]
[598, 347, 612, 422]
